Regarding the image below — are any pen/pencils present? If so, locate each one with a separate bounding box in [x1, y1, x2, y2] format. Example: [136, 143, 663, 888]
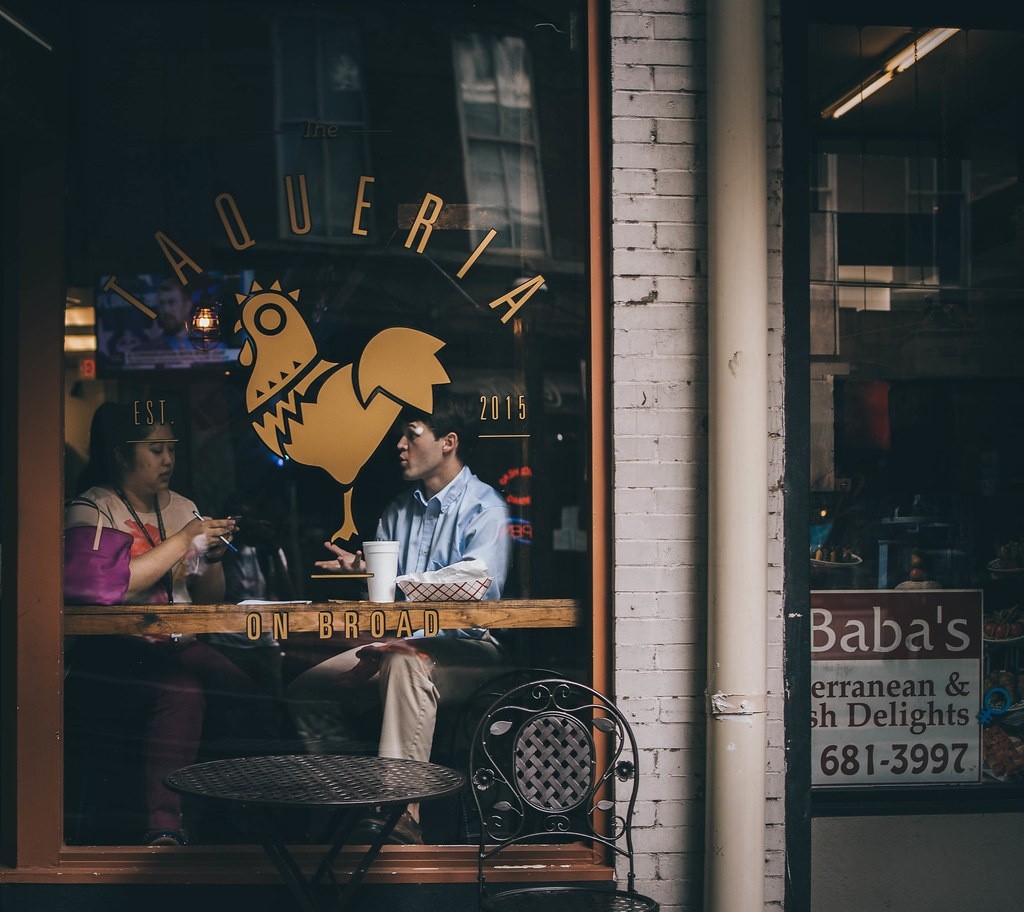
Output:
[192, 510, 238, 553]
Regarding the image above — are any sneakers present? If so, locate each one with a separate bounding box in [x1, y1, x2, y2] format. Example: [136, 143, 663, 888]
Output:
[359, 812, 423, 844]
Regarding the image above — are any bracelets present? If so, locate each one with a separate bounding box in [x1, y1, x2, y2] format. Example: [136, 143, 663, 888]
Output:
[203, 554, 225, 564]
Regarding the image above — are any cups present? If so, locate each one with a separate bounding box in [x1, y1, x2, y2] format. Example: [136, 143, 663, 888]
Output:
[362, 540, 400, 603]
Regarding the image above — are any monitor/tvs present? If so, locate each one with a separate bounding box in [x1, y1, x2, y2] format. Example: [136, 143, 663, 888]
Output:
[95, 269, 287, 379]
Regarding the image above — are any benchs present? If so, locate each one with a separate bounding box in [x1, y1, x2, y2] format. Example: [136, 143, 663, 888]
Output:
[62, 594, 583, 636]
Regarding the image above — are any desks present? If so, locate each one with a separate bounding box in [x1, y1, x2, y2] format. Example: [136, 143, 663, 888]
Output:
[162, 753, 466, 912]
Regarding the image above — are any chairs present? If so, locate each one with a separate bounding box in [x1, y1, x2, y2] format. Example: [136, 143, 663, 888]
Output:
[467, 676, 661, 912]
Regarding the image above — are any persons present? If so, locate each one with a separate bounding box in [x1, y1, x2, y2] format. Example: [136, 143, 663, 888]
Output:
[66, 400, 281, 844]
[283, 386, 514, 844]
[207, 492, 325, 687]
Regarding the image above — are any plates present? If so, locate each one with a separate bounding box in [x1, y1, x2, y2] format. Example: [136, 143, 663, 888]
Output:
[984, 634, 1024, 643]
[809, 553, 862, 568]
[989, 699, 1024, 711]
[986, 558, 1024, 573]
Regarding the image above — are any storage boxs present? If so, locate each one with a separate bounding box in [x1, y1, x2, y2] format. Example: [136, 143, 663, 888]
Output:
[835, 264, 938, 311]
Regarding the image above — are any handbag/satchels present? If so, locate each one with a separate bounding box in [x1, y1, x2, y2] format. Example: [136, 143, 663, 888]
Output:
[63, 495, 136, 602]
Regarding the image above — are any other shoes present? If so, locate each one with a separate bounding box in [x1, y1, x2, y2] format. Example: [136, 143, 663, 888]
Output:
[140, 829, 193, 846]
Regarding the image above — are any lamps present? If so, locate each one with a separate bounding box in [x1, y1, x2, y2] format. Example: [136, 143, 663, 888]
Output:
[821, 28, 961, 122]
[186, 264, 227, 351]
